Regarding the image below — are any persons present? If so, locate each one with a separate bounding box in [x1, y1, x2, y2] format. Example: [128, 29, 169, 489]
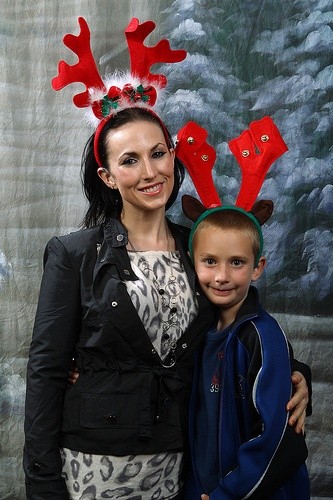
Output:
[22, 15, 220, 500]
[177, 116, 312, 500]
